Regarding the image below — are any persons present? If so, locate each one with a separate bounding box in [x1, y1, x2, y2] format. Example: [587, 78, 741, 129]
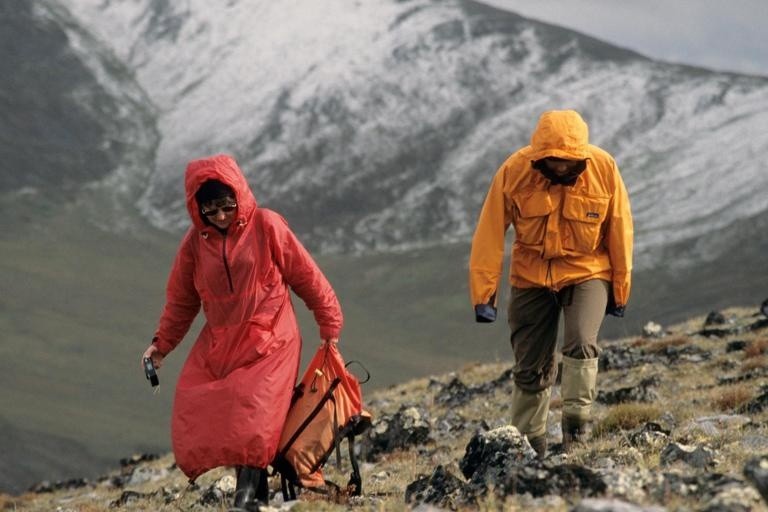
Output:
[141, 154, 345, 512]
[468, 108, 633, 460]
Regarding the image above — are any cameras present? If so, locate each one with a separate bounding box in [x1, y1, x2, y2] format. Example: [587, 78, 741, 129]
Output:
[142, 356, 160, 388]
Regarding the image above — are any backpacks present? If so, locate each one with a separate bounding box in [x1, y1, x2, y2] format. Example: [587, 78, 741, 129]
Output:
[276, 340, 370, 491]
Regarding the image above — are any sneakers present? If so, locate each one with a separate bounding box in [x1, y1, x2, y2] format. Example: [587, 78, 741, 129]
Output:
[528, 432, 546, 462]
[234, 469, 268, 508]
[561, 426, 586, 454]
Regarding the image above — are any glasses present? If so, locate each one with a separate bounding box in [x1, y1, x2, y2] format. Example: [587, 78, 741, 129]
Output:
[202, 203, 236, 216]
